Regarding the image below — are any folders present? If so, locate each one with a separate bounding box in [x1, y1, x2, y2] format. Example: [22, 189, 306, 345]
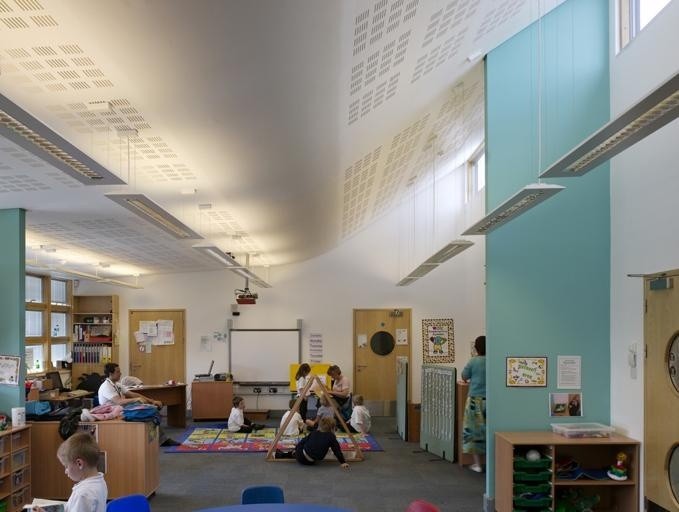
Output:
[73, 344, 103, 363]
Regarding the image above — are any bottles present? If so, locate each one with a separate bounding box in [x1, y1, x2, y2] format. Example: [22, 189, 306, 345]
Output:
[35, 359, 40, 369]
[52, 324, 59, 337]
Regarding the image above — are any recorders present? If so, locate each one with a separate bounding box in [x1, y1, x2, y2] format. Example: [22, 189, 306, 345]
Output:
[214, 373, 233, 381]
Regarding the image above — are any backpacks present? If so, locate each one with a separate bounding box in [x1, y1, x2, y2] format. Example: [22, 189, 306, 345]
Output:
[123, 403, 159, 425]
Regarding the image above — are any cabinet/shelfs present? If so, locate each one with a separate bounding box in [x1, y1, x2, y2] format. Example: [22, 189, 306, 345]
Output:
[495, 431, 642, 512]
[73, 294, 119, 390]
[0, 424, 32, 511]
[24, 271, 73, 376]
[192, 381, 233, 421]
[27, 419, 160, 499]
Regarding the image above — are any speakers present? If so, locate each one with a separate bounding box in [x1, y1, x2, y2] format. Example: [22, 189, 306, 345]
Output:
[232, 311, 240, 316]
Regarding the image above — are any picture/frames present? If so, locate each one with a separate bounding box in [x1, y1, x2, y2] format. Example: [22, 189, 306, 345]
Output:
[506, 356, 547, 386]
[549, 393, 583, 416]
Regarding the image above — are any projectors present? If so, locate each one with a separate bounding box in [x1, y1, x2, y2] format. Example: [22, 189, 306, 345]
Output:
[239, 293, 258, 298]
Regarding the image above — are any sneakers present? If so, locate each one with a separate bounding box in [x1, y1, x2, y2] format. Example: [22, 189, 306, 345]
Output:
[275, 447, 283, 459]
[255, 424, 264, 430]
[160, 438, 181, 447]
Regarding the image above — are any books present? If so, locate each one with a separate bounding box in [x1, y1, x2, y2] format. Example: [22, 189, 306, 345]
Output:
[74, 325, 112, 365]
[22, 497, 68, 512]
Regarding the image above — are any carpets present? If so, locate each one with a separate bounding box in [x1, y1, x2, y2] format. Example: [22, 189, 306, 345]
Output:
[164, 423, 385, 452]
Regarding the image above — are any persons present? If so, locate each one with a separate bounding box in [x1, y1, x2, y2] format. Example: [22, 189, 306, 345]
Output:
[327, 365, 350, 407]
[306, 395, 334, 432]
[279, 399, 305, 437]
[337, 395, 371, 438]
[98, 363, 181, 445]
[228, 396, 265, 433]
[461, 336, 486, 472]
[33, 433, 109, 511]
[276, 415, 349, 468]
[295, 363, 313, 422]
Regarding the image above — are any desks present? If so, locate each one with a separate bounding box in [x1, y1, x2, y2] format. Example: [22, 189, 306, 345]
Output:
[194, 503, 352, 512]
[39, 391, 95, 412]
[128, 383, 188, 429]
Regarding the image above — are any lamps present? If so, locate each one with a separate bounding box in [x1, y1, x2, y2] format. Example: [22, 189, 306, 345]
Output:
[25, 243, 144, 290]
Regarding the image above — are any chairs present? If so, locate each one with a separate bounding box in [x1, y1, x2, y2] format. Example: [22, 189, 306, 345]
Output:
[241, 485, 284, 504]
[106, 495, 152, 512]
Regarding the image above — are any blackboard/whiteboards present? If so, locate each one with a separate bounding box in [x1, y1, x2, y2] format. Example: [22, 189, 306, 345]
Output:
[229, 329, 302, 386]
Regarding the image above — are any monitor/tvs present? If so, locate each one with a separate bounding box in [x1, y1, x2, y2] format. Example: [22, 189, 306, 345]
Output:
[46, 372, 63, 391]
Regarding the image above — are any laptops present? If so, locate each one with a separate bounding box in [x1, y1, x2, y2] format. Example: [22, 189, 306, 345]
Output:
[195, 360, 214, 377]
